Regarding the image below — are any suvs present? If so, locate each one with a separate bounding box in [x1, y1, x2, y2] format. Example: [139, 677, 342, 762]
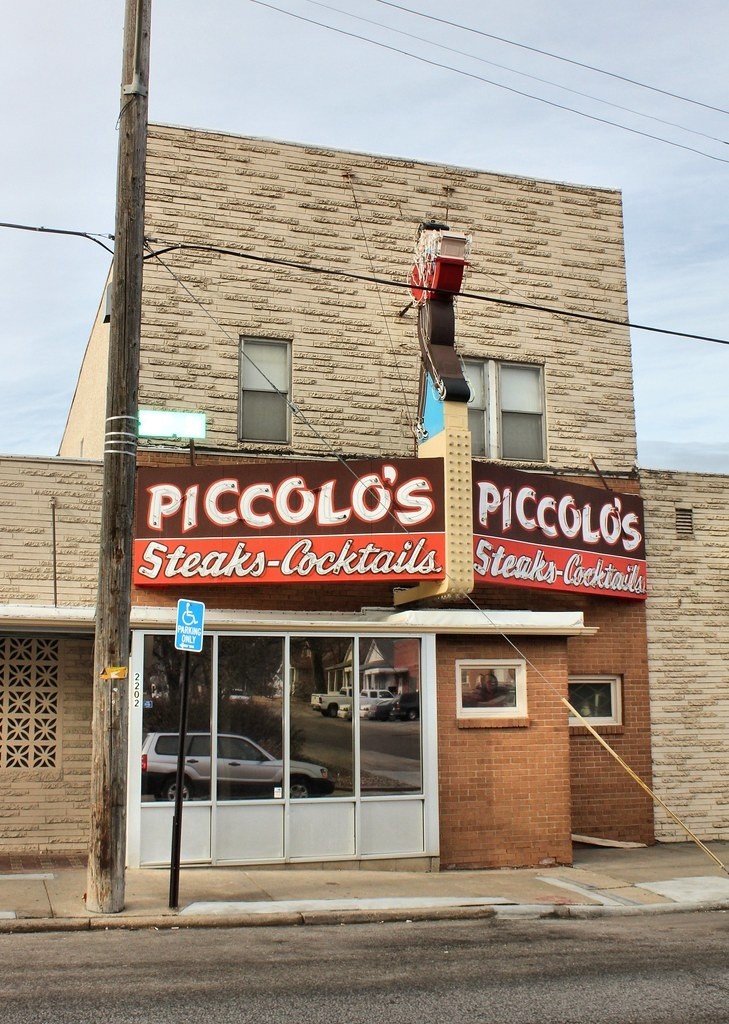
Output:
[137, 731, 334, 802]
[390, 691, 420, 721]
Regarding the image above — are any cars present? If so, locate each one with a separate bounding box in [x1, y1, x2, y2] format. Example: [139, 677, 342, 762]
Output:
[221, 687, 252, 705]
[367, 698, 396, 721]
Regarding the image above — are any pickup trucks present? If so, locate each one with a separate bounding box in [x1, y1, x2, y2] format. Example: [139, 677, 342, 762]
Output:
[312, 684, 354, 717]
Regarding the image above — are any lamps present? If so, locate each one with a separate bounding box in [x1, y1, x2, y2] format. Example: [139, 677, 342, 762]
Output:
[138, 408, 207, 462]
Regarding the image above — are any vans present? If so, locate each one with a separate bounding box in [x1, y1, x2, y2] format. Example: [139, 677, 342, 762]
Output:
[362, 689, 397, 708]
